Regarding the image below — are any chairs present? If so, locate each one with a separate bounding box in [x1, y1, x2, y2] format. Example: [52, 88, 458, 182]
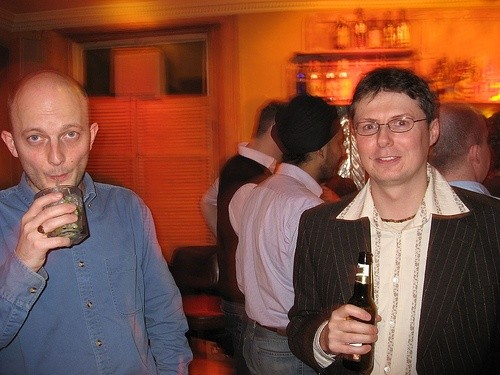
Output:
[170, 245, 226, 342]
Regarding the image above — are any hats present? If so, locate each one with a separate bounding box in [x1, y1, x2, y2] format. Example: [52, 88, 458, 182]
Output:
[270, 95, 341, 154]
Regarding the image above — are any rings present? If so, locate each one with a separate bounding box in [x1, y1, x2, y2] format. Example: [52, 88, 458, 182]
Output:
[37, 225, 45, 234]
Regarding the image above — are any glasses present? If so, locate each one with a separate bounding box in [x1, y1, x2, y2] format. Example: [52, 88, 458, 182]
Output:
[354, 118, 428, 136]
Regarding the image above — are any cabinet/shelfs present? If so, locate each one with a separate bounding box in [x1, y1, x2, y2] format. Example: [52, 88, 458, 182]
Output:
[70, 28, 217, 262]
[291, 49, 500, 110]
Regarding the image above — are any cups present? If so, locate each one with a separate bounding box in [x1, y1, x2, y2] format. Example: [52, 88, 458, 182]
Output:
[34, 185, 88, 245]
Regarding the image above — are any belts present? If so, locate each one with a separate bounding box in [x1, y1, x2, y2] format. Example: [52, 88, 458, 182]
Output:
[252, 320, 280, 331]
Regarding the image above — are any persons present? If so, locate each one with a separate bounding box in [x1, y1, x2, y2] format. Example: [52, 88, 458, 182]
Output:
[236, 94, 343, 375]
[204, 101, 371, 375]
[428, 103, 500, 200]
[288, 67, 500, 375]
[0, 69, 192, 375]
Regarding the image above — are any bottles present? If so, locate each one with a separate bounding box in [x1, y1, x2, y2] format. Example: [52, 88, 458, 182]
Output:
[293, 59, 355, 101]
[333, 6, 412, 51]
[343, 251, 377, 371]
